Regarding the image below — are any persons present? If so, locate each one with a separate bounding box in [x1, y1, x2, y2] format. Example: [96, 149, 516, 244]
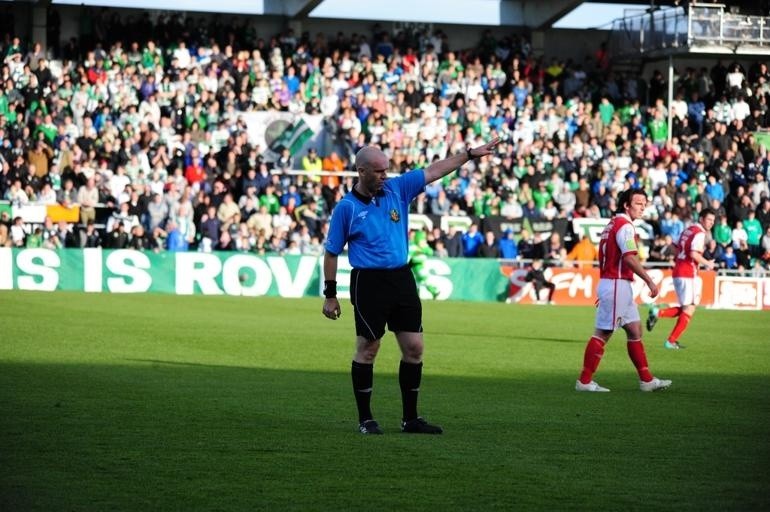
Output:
[321, 46, 770, 275]
[646, 208, 716, 350]
[2, 34, 321, 254]
[321, 138, 501, 434]
[575, 187, 673, 394]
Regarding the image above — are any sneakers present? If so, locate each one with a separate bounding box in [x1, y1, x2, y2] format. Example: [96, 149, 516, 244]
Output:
[664, 340, 686, 350]
[358, 419, 383, 435]
[639, 376, 672, 392]
[645, 307, 660, 332]
[575, 380, 610, 392]
[400, 416, 443, 435]
